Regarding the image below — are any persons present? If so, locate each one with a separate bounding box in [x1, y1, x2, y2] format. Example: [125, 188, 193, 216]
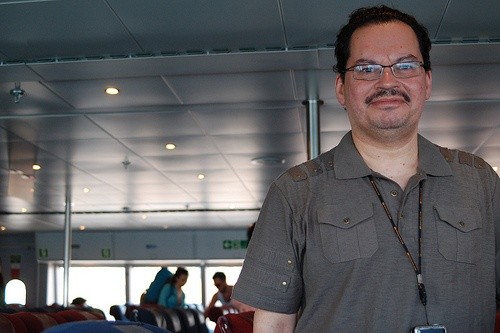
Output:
[159, 267, 189, 307]
[205, 272, 234, 314]
[231, 4, 500, 333]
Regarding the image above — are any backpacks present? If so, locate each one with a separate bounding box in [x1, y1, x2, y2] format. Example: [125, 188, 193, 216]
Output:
[141, 267, 174, 309]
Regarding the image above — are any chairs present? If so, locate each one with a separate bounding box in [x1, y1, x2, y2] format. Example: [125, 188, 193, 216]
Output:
[0, 305, 254, 333]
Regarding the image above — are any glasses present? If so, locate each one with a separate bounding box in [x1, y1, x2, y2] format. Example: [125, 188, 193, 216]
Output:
[344, 61, 426, 81]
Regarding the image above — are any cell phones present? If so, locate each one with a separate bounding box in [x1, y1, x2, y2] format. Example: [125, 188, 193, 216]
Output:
[411, 324, 448, 333]
